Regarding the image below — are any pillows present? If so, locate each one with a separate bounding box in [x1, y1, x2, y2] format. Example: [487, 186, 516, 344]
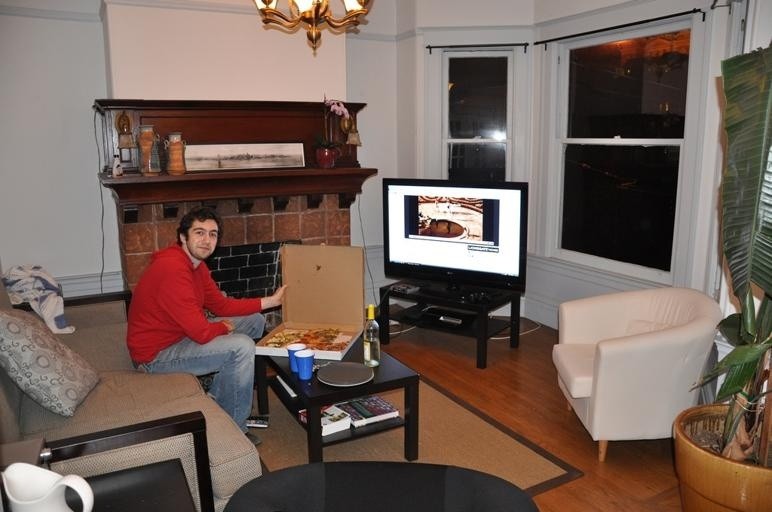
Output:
[0, 308, 100, 418]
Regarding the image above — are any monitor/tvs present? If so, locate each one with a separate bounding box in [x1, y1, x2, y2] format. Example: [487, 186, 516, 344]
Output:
[382, 177, 529, 300]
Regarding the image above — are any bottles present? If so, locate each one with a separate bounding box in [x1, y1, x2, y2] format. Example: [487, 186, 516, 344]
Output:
[148, 139, 161, 174]
[362, 301, 381, 369]
[111, 153, 125, 178]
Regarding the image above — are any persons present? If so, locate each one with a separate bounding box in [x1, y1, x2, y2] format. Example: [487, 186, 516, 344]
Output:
[126, 206, 288, 447]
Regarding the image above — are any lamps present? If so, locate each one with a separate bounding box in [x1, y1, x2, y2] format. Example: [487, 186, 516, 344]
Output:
[342, 114, 362, 146]
[115, 111, 137, 150]
[253, 0, 370, 56]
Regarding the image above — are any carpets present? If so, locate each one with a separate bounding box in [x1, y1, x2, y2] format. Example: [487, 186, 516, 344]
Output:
[247, 367, 583, 497]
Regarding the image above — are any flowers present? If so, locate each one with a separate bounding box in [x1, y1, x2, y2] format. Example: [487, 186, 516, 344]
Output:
[321, 94, 349, 147]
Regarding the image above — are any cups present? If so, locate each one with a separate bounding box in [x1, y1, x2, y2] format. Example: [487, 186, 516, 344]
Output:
[293, 350, 316, 381]
[286, 342, 306, 373]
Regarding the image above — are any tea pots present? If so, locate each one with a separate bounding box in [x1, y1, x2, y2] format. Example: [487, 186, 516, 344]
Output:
[0, 461, 96, 512]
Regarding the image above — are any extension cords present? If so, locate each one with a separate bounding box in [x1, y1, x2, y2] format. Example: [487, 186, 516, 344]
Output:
[92, 102, 105, 116]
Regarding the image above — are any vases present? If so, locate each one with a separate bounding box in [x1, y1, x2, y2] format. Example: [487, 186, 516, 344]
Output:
[314, 145, 342, 168]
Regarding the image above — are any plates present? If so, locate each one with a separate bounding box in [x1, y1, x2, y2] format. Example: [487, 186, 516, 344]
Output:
[314, 361, 379, 388]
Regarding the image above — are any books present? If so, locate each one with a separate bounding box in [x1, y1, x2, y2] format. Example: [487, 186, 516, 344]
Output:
[335, 395, 399, 427]
[298, 405, 351, 435]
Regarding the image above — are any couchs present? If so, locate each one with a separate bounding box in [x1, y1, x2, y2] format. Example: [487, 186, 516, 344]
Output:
[0, 277, 263, 512]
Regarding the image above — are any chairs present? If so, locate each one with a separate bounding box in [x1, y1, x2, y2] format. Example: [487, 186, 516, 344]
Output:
[552, 287, 722, 464]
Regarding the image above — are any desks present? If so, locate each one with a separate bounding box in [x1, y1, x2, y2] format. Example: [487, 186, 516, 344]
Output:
[378, 281, 522, 370]
[65, 458, 198, 512]
[223, 461, 539, 512]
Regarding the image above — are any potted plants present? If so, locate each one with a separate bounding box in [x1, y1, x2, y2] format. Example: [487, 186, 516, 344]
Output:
[672, 43, 772, 512]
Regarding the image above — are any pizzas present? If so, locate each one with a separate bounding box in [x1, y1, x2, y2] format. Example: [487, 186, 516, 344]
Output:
[264, 326, 350, 351]
